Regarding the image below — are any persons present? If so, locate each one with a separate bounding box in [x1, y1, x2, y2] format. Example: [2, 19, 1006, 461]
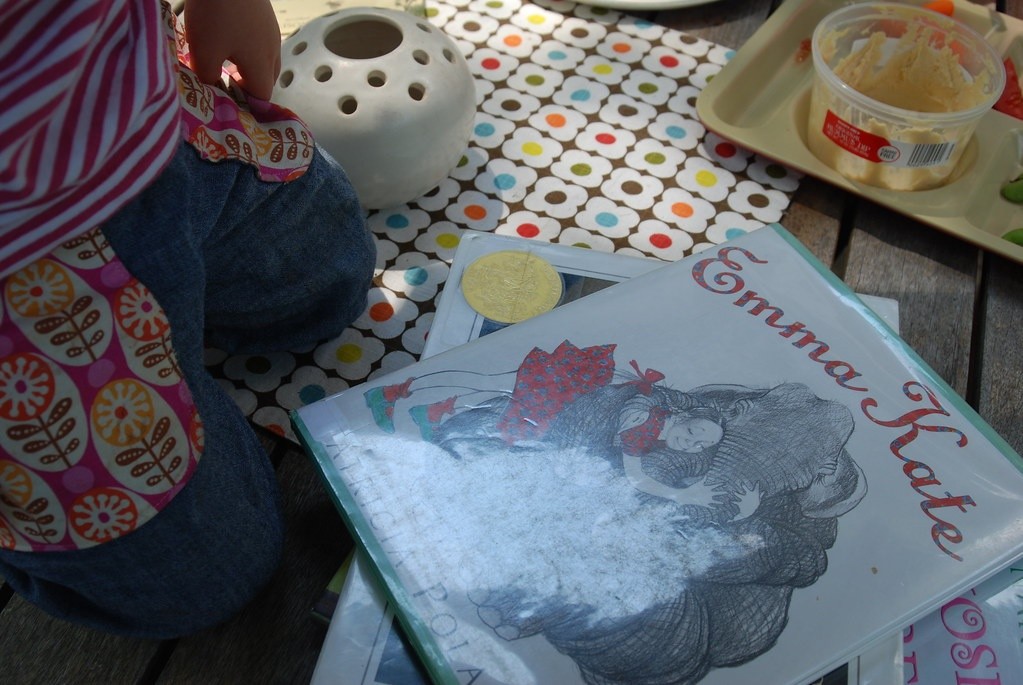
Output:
[0, 0, 374, 633]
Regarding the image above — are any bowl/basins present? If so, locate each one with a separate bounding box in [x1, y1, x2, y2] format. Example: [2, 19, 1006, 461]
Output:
[809, 2, 1006, 190]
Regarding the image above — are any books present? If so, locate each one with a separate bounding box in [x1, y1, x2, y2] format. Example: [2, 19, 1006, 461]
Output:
[293, 222, 1023, 684]
